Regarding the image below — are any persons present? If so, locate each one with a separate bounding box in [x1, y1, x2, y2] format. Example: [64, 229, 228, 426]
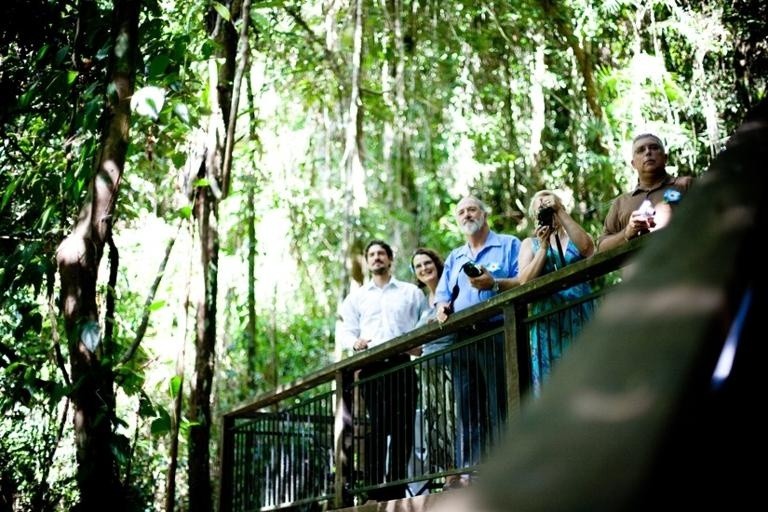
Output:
[338, 241, 432, 501]
[597, 133, 694, 254]
[436, 196, 522, 490]
[517, 190, 595, 407]
[409, 248, 461, 490]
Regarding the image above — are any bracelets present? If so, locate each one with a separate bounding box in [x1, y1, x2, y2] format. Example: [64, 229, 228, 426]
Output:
[621, 225, 630, 244]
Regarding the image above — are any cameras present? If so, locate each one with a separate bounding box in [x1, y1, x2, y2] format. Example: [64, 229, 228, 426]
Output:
[536, 206, 555, 232]
[463, 262, 482, 277]
[639, 199, 656, 222]
